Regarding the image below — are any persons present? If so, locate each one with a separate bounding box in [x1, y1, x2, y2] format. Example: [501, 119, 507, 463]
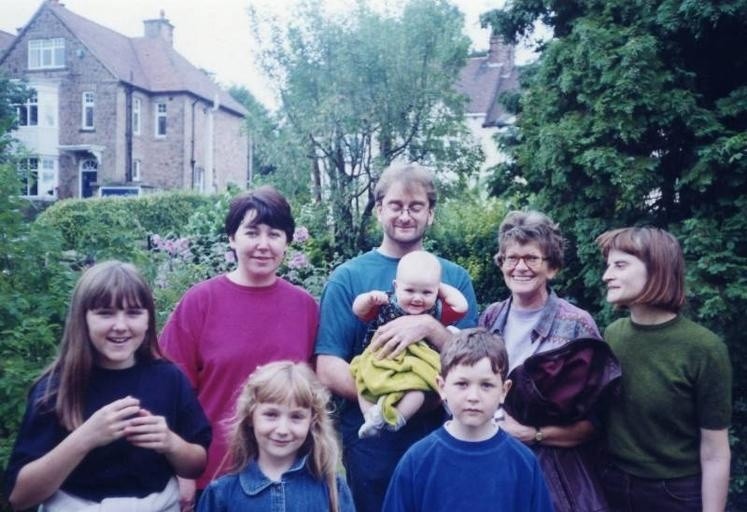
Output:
[195, 359, 356, 512]
[352, 249, 471, 440]
[153, 184, 320, 511]
[313, 163, 481, 510]
[475, 209, 623, 512]
[0, 260, 214, 512]
[592, 225, 732, 512]
[379, 325, 554, 511]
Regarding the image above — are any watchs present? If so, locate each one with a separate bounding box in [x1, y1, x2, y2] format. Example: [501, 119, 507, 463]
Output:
[534, 424, 543, 445]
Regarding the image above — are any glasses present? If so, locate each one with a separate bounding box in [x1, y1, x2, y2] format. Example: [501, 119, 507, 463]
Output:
[503, 254, 551, 268]
